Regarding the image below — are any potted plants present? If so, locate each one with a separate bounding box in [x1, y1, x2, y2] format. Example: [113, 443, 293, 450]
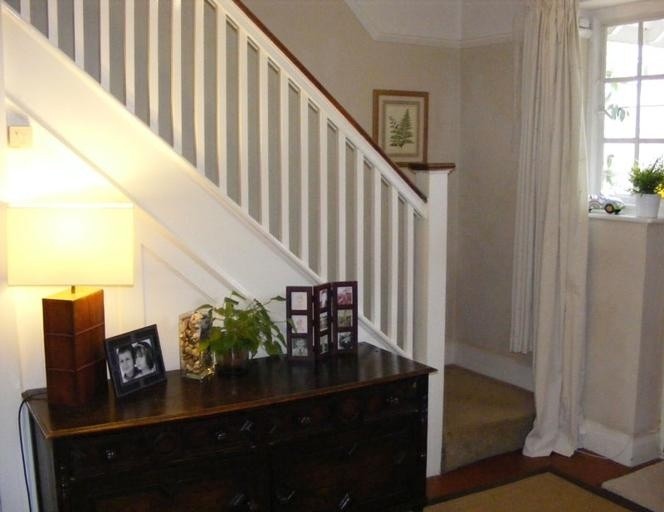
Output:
[623, 156, 664, 219]
[189, 290, 298, 375]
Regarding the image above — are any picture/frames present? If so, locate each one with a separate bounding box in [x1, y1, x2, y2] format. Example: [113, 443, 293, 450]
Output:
[372, 88, 429, 168]
[286, 281, 359, 361]
[103, 324, 167, 399]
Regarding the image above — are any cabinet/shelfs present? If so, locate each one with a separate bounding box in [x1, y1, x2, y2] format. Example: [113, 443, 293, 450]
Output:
[21, 340, 438, 512]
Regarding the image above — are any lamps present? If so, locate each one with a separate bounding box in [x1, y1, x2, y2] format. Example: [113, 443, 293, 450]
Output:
[0, 194, 138, 423]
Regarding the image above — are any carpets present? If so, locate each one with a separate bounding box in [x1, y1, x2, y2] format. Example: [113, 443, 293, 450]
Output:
[416, 466, 655, 512]
[600, 461, 664, 512]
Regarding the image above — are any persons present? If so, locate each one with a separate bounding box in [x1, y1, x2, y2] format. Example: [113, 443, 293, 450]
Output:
[293, 338, 308, 358]
[131, 340, 156, 380]
[317, 287, 329, 309]
[117, 344, 138, 381]
